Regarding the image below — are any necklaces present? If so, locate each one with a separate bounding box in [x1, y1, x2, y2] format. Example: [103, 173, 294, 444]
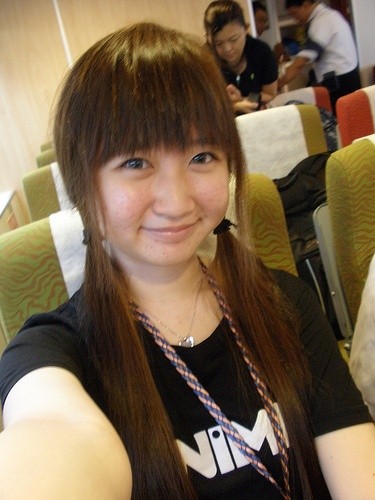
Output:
[120, 273, 204, 348]
[230, 64, 246, 81]
[120, 254, 291, 500]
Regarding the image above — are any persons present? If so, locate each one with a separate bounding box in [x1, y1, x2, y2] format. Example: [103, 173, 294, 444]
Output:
[0, 22, 375, 500]
[252, 0, 362, 119]
[202, 0, 278, 117]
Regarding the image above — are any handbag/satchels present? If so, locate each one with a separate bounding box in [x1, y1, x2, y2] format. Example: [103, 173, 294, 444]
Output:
[264, 151, 357, 258]
[285, 99, 339, 151]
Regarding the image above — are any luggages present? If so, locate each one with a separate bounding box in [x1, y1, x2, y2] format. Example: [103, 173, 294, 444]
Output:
[294, 248, 345, 341]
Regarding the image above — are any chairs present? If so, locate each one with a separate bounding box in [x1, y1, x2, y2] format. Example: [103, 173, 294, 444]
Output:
[0, 81, 375, 361]
